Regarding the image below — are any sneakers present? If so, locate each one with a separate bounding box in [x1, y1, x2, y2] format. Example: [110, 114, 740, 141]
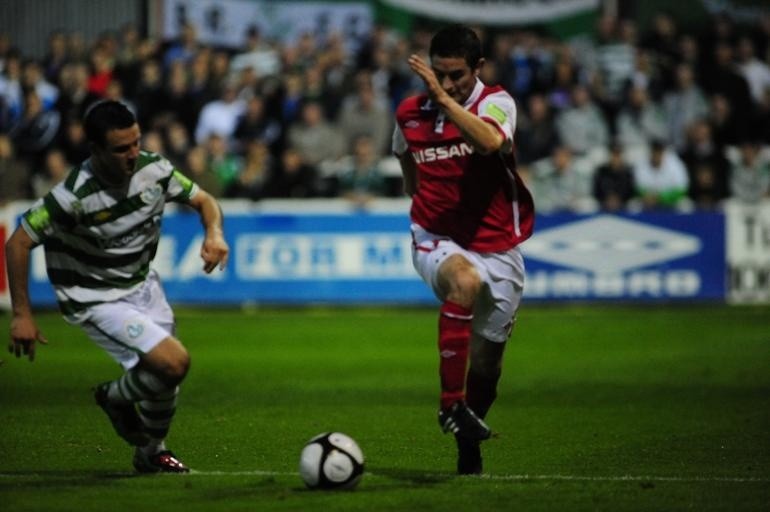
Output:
[94, 381, 150, 448]
[438, 399, 491, 439]
[455, 439, 481, 475]
[133, 448, 190, 474]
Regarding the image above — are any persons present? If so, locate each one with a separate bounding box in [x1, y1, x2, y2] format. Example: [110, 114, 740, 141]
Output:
[391, 25, 534, 476]
[0, 9, 770, 214]
[5, 99, 229, 474]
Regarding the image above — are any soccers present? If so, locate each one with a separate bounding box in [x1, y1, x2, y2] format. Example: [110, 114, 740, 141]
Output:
[300, 432, 364, 490]
